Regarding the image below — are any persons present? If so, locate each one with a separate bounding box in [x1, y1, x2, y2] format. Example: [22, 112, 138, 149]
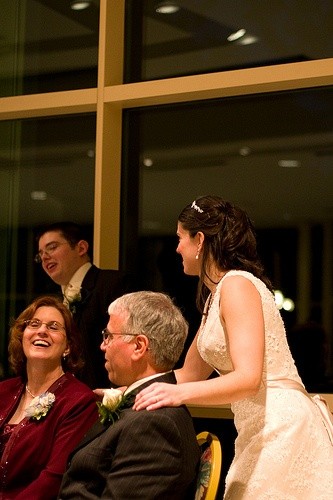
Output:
[93, 196, 333, 500]
[38, 222, 132, 390]
[63, 291, 201, 500]
[0, 297, 99, 500]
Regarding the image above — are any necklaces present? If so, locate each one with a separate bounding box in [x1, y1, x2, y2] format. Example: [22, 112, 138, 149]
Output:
[26, 385, 35, 398]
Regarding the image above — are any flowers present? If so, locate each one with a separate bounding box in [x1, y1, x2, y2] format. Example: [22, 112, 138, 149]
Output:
[25, 393, 55, 421]
[94, 388, 135, 433]
[64, 284, 82, 314]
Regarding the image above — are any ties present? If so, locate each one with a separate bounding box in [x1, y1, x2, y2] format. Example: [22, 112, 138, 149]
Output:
[63, 284, 71, 310]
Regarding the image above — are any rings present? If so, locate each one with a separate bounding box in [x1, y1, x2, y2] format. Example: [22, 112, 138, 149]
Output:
[155, 395, 160, 402]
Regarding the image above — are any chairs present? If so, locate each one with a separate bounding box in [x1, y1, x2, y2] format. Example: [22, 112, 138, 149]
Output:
[195, 431, 222, 500]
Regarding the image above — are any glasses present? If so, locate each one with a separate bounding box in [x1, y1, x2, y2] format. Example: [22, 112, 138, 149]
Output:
[24, 319, 65, 332]
[102, 329, 149, 352]
[35, 241, 79, 263]
